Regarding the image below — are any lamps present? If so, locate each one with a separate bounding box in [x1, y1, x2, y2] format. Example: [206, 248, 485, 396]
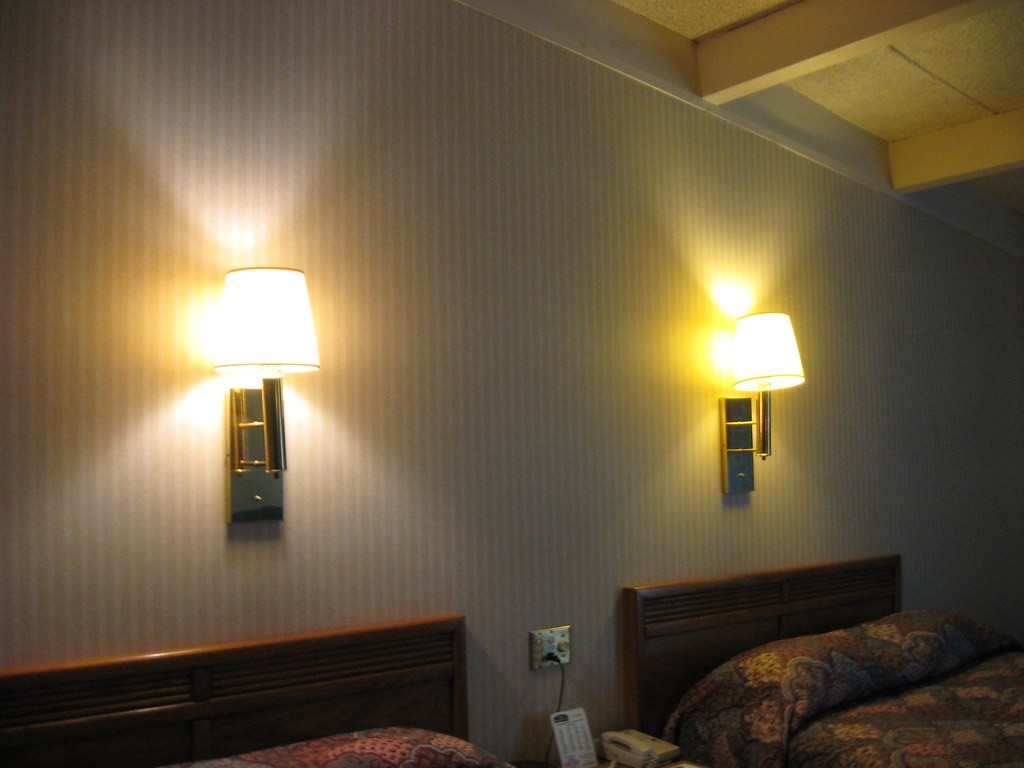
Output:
[211, 266, 322, 526]
[717, 313, 806, 494]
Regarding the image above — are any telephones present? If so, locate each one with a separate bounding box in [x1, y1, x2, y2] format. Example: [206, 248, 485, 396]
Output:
[593, 729, 680, 768]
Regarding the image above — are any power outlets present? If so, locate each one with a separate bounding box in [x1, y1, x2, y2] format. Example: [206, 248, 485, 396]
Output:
[530, 626, 570, 668]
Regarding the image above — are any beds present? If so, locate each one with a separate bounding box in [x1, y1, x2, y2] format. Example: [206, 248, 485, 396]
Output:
[0, 615, 513, 768]
[618, 556, 1024, 768]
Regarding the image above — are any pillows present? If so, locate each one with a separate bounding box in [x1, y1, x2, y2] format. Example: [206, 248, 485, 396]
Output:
[710, 610, 996, 718]
[171, 726, 511, 768]
